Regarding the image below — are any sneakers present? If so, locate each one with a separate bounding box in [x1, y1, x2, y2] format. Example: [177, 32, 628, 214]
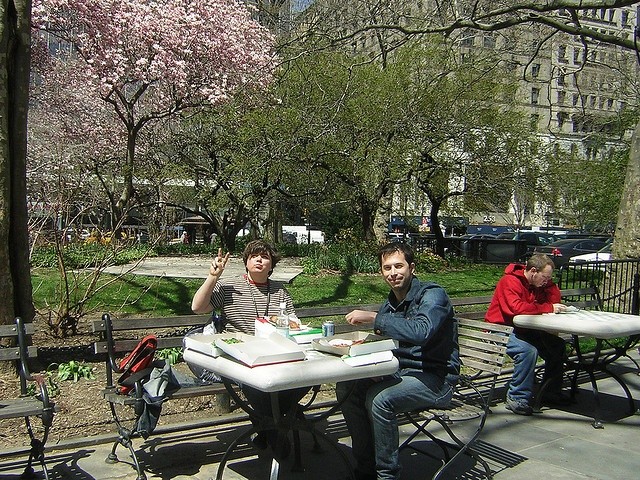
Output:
[504, 391, 531, 415]
[249, 414, 268, 448]
[269, 431, 290, 461]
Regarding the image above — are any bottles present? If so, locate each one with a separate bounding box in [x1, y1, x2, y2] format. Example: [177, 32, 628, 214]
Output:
[276, 302, 290, 338]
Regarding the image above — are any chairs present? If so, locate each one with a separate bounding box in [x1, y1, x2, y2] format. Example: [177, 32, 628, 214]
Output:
[400, 314, 514, 480]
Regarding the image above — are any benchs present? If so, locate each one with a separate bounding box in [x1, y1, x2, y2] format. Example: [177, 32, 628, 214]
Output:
[1, 317, 57, 479]
[88, 283, 635, 479]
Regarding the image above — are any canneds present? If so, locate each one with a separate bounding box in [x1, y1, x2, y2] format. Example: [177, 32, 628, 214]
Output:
[322, 321, 335, 338]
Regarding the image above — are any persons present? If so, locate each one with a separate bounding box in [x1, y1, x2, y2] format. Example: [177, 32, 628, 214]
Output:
[190, 240, 303, 459]
[333, 241, 462, 479]
[481, 253, 572, 416]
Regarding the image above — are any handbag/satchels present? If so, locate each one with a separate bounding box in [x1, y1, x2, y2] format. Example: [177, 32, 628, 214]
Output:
[114, 335, 158, 395]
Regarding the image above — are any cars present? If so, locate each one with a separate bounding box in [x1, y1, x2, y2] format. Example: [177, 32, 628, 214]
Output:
[536, 238, 607, 268]
[63, 229, 91, 239]
[86, 231, 136, 242]
[568, 242, 613, 271]
[463, 235, 494, 244]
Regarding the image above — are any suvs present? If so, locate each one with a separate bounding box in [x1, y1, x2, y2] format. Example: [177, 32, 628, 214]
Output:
[495, 231, 551, 255]
